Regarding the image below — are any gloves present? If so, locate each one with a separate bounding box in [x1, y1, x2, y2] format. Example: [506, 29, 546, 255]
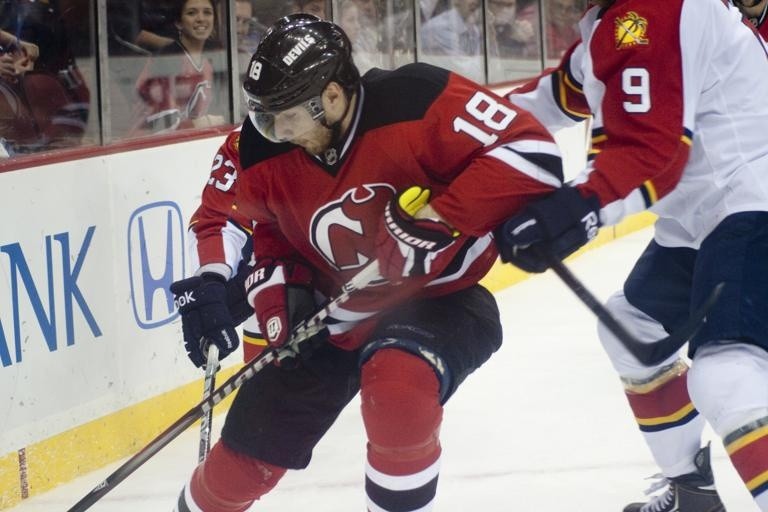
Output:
[245, 254, 322, 365]
[170, 263, 239, 368]
[494, 185, 600, 275]
[374, 185, 459, 284]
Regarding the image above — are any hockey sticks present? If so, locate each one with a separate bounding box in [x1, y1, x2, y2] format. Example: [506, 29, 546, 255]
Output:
[543, 242, 727, 366]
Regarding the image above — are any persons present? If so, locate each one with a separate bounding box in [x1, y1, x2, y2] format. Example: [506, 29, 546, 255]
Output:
[168, 12, 566, 512]
[2, 1, 255, 156]
[495, 0, 767, 509]
[731, 0, 768, 43]
[298, 0, 591, 85]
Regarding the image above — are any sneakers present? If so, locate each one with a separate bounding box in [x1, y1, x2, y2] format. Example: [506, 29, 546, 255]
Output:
[623, 441, 726, 512]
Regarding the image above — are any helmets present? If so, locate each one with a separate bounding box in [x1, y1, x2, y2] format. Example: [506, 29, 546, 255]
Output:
[241, 12, 352, 142]
